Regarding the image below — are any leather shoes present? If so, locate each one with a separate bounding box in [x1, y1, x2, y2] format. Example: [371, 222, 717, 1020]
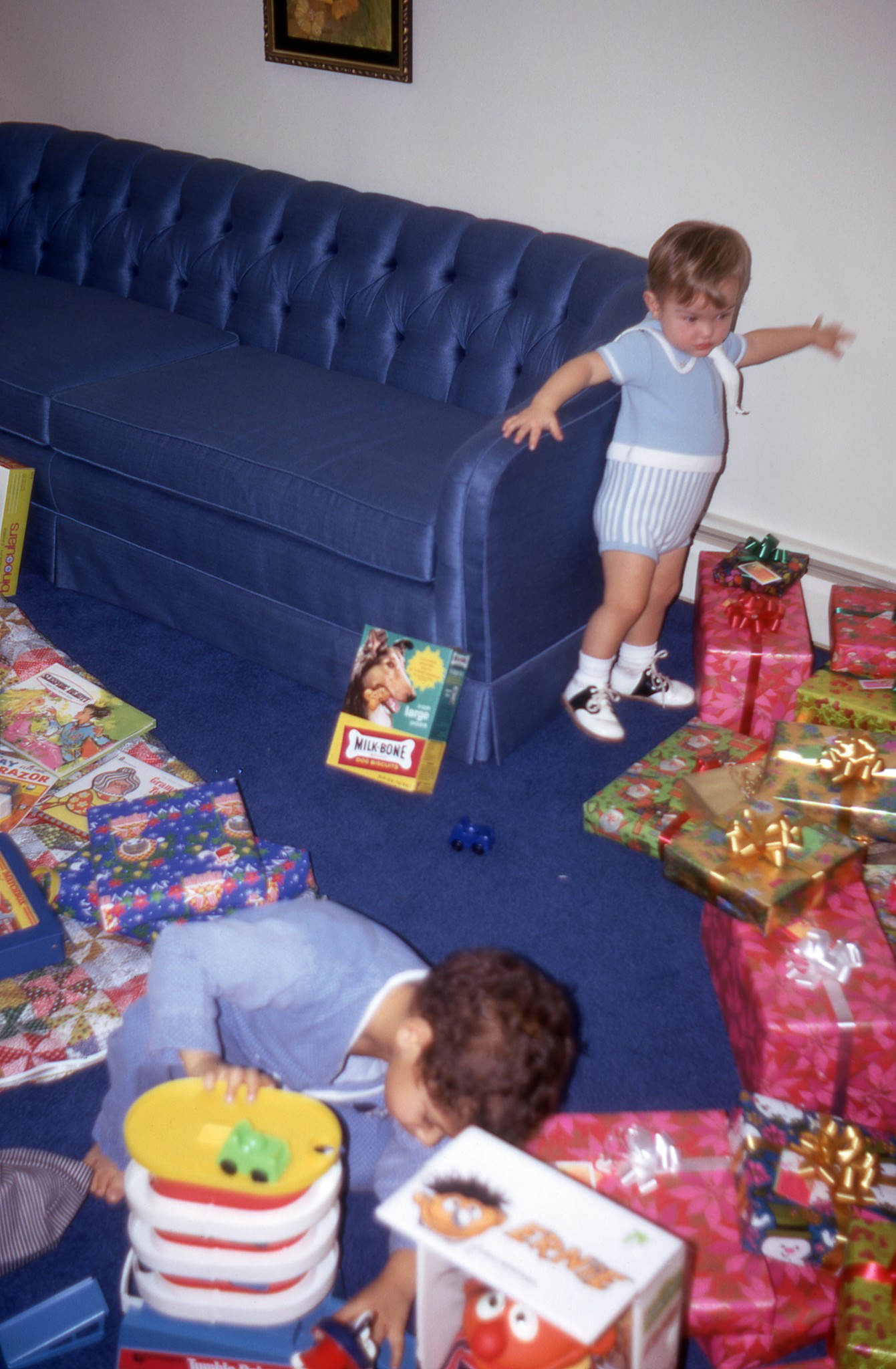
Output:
[562, 679, 625, 742]
[611, 652, 696, 707]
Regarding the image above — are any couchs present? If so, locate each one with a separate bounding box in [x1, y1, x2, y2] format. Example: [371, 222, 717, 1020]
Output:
[0, 121, 648, 764]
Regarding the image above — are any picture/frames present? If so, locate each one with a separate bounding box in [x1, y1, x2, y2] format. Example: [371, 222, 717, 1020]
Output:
[263, 0, 413, 84]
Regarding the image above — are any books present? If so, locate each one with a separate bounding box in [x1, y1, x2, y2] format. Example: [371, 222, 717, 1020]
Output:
[0, 662, 195, 833]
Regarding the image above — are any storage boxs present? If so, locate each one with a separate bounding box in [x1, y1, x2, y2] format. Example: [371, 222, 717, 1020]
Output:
[0, 833, 66, 981]
[699, 878, 896, 1142]
[828, 585, 896, 682]
[88, 779, 266, 935]
[797, 661, 896, 735]
[374, 1125, 684, 1369]
[696, 552, 813, 737]
[526, 1109, 896, 1368]
[324, 624, 471, 795]
[663, 795, 867, 936]
[681, 758, 763, 815]
[0, 456, 35, 597]
[582, 716, 766, 857]
[761, 720, 896, 840]
[42, 834, 316, 943]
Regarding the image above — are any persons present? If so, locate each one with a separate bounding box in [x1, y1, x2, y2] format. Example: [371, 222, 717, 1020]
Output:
[500, 219, 853, 744]
[90, 898, 589, 1369]
